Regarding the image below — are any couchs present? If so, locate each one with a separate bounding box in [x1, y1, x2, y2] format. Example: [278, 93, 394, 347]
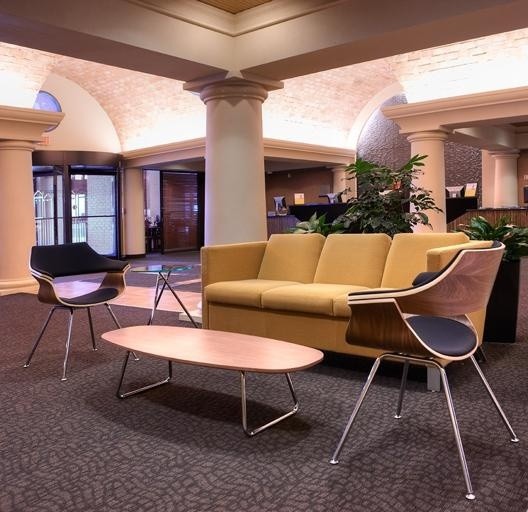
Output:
[198, 232, 505, 384]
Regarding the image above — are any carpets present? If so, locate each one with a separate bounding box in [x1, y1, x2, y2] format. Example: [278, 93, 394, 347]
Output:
[80, 250, 203, 292]
[1, 291, 528, 510]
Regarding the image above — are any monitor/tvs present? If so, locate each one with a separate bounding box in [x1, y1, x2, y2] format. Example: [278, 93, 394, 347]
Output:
[445, 186, 464, 197]
[272, 196, 287, 215]
[521, 185, 528, 206]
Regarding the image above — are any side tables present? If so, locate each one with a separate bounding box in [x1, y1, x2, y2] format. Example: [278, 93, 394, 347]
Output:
[130, 264, 198, 327]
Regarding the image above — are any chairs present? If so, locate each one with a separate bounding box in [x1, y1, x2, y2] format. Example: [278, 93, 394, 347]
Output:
[330, 241, 521, 499]
[24, 240, 133, 382]
